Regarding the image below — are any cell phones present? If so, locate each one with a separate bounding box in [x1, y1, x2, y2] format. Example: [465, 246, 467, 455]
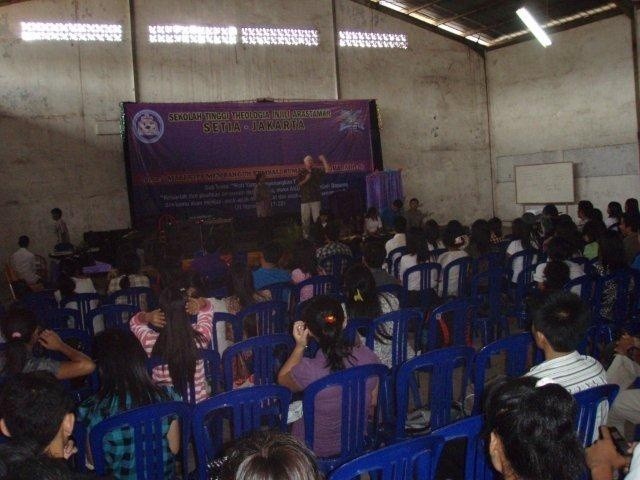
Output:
[609, 426, 631, 456]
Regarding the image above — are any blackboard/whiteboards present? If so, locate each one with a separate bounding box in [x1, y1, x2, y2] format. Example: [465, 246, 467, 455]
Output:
[514, 162, 574, 204]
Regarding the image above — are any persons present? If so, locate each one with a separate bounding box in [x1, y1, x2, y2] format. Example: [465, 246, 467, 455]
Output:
[253, 173, 277, 243]
[295, 154, 332, 238]
[0, 197, 640, 480]
